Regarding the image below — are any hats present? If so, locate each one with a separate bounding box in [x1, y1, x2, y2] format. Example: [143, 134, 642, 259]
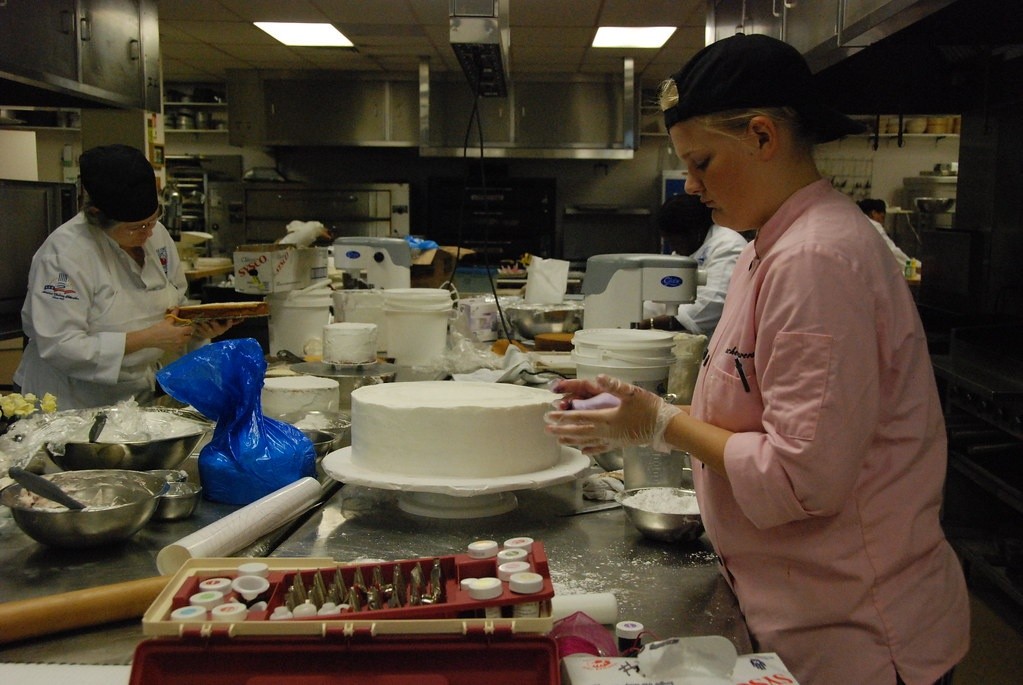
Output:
[79, 143, 159, 224]
[664, 33, 869, 144]
[655, 191, 708, 240]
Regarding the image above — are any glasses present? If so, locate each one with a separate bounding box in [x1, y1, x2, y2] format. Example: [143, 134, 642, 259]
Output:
[117, 206, 165, 234]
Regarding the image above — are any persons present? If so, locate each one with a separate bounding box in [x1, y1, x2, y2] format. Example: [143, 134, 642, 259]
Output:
[13, 143, 244, 412]
[640, 195, 748, 340]
[858, 198, 921, 268]
[542, 32, 970, 685]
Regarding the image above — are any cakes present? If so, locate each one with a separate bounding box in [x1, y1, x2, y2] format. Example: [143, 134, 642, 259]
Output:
[258, 374, 339, 417]
[322, 322, 377, 364]
[350, 380, 566, 482]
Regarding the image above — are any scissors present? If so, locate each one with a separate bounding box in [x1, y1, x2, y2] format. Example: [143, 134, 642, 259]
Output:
[164, 313, 208, 327]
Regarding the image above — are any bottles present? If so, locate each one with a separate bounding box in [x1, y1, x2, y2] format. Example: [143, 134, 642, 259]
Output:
[910, 258, 916, 279]
[905, 261, 912, 277]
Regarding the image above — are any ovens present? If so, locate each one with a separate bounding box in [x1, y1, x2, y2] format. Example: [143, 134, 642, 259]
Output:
[207, 182, 410, 257]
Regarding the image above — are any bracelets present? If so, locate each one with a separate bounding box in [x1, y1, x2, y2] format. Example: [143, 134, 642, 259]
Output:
[651, 318, 654, 327]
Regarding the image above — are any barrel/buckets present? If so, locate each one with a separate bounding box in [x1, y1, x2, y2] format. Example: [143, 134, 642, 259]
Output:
[571, 328, 678, 397]
[263, 288, 453, 361]
[665, 331, 708, 405]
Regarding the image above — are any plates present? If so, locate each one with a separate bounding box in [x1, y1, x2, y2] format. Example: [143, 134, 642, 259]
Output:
[881, 116, 960, 134]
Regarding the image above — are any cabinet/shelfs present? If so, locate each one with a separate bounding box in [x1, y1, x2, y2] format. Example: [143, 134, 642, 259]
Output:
[0, 0, 141, 110]
[706, 0, 996, 89]
[163, 81, 227, 133]
[225, 69, 635, 161]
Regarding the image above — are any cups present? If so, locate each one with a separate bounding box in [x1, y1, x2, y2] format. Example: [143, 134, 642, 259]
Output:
[624, 444, 684, 491]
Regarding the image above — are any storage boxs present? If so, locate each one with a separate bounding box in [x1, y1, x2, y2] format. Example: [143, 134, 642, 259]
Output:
[233, 244, 314, 296]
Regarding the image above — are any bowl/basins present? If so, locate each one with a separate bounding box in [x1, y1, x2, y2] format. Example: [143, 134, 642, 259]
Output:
[151, 482, 203, 522]
[146, 470, 189, 483]
[0, 469, 170, 551]
[614, 487, 704, 543]
[298, 429, 340, 457]
[913, 197, 955, 213]
[504, 303, 583, 341]
[42, 406, 212, 473]
[593, 446, 624, 472]
[275, 409, 352, 441]
